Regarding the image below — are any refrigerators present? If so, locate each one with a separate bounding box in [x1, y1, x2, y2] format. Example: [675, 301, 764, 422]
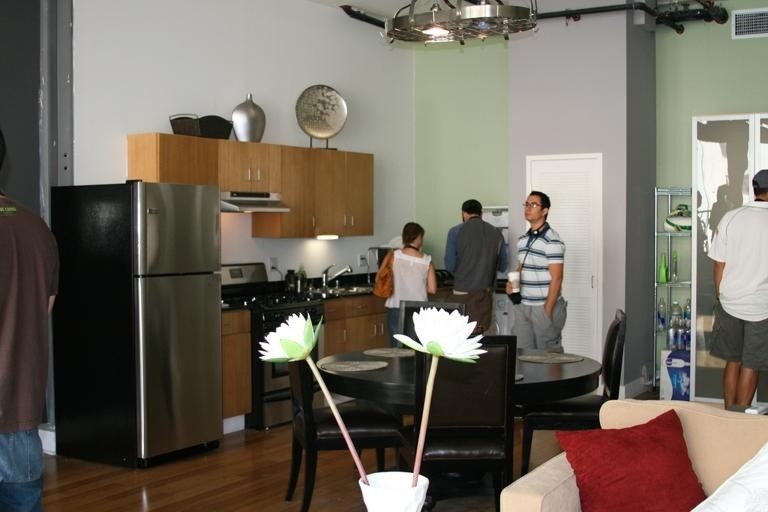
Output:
[47, 182, 223, 471]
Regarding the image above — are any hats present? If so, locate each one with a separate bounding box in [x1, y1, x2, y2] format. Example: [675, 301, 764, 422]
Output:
[753, 170, 768, 189]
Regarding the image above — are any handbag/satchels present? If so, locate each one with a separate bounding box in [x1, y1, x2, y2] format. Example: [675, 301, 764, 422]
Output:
[374, 262, 393, 298]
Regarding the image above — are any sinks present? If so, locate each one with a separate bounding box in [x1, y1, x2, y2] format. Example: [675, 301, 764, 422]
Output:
[314, 286, 373, 296]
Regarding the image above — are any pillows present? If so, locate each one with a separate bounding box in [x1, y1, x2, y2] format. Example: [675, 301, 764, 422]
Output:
[553, 409, 709, 512]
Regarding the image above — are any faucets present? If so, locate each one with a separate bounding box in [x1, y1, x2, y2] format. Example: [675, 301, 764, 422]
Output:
[321, 263, 353, 287]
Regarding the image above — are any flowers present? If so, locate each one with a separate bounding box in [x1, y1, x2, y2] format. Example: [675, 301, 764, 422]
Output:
[258, 307, 488, 486]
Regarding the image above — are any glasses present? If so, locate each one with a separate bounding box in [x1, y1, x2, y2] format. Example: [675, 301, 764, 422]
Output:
[523, 202, 540, 209]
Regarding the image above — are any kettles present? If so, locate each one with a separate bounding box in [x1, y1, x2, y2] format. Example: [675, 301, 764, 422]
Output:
[284, 269, 296, 295]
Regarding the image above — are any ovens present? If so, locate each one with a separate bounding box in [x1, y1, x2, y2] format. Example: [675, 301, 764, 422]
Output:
[256, 320, 327, 425]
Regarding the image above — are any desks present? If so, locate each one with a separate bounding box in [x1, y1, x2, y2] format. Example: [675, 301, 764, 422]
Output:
[313, 344, 602, 426]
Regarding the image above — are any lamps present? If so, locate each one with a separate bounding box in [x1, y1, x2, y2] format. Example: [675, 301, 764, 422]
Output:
[378, 2, 539, 48]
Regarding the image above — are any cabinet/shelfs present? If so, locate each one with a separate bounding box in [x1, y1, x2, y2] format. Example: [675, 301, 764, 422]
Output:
[219, 310, 253, 418]
[281, 146, 316, 238]
[692, 113, 768, 402]
[128, 132, 219, 240]
[344, 294, 389, 353]
[321, 299, 343, 356]
[316, 149, 374, 237]
[652, 185, 691, 398]
[218, 138, 280, 238]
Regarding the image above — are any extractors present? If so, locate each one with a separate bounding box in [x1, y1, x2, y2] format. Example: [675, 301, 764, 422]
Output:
[219, 190, 291, 214]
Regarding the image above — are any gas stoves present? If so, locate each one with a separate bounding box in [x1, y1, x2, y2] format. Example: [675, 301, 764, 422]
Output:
[233, 289, 325, 318]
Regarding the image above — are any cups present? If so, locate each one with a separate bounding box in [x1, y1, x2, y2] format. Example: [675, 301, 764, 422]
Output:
[508, 271, 520, 293]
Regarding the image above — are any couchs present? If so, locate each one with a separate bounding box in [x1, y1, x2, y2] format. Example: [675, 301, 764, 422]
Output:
[498, 394, 767, 511]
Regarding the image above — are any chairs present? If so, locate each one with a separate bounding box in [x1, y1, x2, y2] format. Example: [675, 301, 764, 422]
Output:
[414, 334, 517, 512]
[521, 309, 628, 478]
[400, 299, 467, 351]
[283, 358, 408, 511]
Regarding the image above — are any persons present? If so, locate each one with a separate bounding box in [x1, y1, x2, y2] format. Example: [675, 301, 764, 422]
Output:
[0, 126, 64, 511]
[707, 168, 768, 412]
[445, 198, 509, 336]
[505, 189, 568, 353]
[375, 222, 439, 350]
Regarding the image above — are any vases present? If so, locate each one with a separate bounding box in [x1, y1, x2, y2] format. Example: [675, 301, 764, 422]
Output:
[353, 473, 430, 512]
[231, 92, 267, 142]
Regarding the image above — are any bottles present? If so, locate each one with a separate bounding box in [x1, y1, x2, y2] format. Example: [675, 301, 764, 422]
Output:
[296, 262, 308, 296]
[670, 251, 680, 282]
[658, 297, 667, 331]
[667, 298, 691, 351]
[658, 253, 666, 283]
